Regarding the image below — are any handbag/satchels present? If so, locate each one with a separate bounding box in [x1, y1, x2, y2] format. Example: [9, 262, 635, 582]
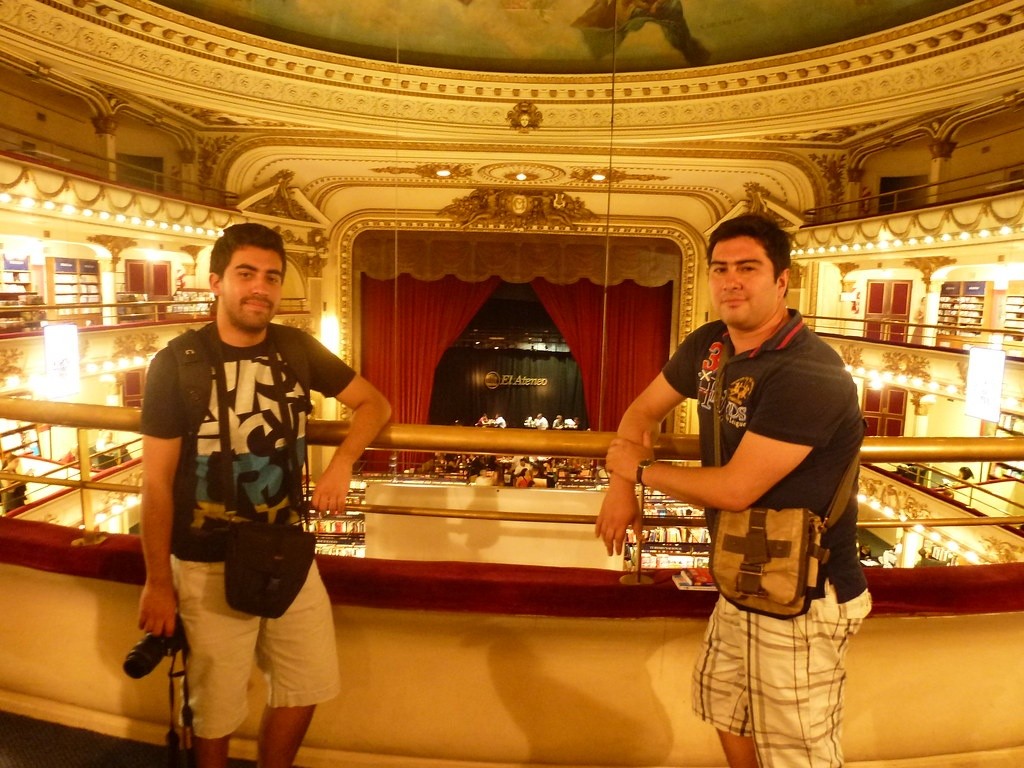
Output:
[223, 521, 317, 619]
[707, 506, 830, 618]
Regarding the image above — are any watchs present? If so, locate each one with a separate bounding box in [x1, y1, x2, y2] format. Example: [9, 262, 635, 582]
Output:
[636, 459, 657, 485]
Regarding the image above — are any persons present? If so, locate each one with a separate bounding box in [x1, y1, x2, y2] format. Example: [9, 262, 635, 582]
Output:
[596, 212, 872, 768]
[909, 297, 926, 344]
[65, 446, 80, 468]
[0, 454, 29, 514]
[535, 414, 549, 430]
[952, 467, 975, 486]
[96, 433, 131, 469]
[573, 417, 582, 430]
[492, 413, 506, 428]
[945, 489, 955, 498]
[554, 415, 565, 429]
[478, 414, 490, 428]
[139, 222, 390, 767]
[860, 544, 871, 559]
[422, 453, 609, 490]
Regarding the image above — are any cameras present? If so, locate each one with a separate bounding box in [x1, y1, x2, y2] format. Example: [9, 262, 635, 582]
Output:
[123, 615, 186, 679]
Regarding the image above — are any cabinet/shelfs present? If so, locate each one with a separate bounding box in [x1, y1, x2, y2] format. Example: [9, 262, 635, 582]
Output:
[45, 256, 103, 328]
[935, 280, 1024, 357]
[984, 408, 1024, 484]
